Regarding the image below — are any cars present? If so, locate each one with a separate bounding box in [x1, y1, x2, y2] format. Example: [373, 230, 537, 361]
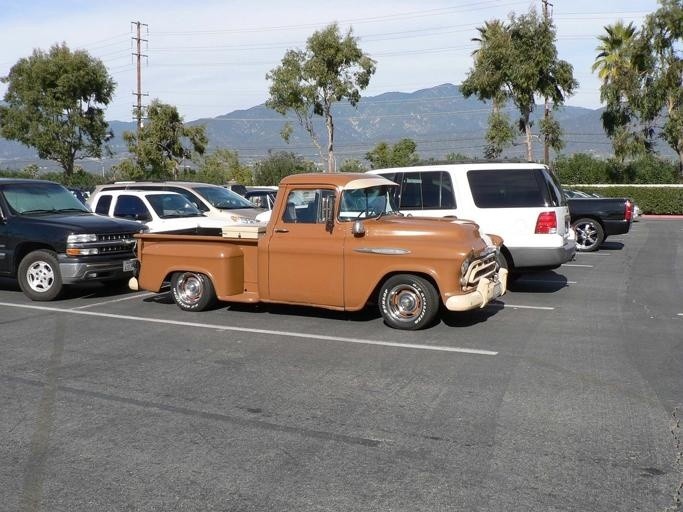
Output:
[562, 188, 641, 222]
[221, 184, 310, 211]
[90, 189, 244, 233]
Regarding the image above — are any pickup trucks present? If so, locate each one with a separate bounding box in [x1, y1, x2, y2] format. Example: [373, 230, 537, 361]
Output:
[128, 171, 509, 332]
[565, 197, 634, 252]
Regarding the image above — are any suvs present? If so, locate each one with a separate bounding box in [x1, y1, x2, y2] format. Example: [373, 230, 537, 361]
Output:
[254, 163, 578, 287]
[84, 181, 272, 224]
[0, 177, 148, 301]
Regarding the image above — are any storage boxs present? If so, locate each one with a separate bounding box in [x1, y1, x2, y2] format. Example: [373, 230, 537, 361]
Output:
[222, 226, 266, 239]
